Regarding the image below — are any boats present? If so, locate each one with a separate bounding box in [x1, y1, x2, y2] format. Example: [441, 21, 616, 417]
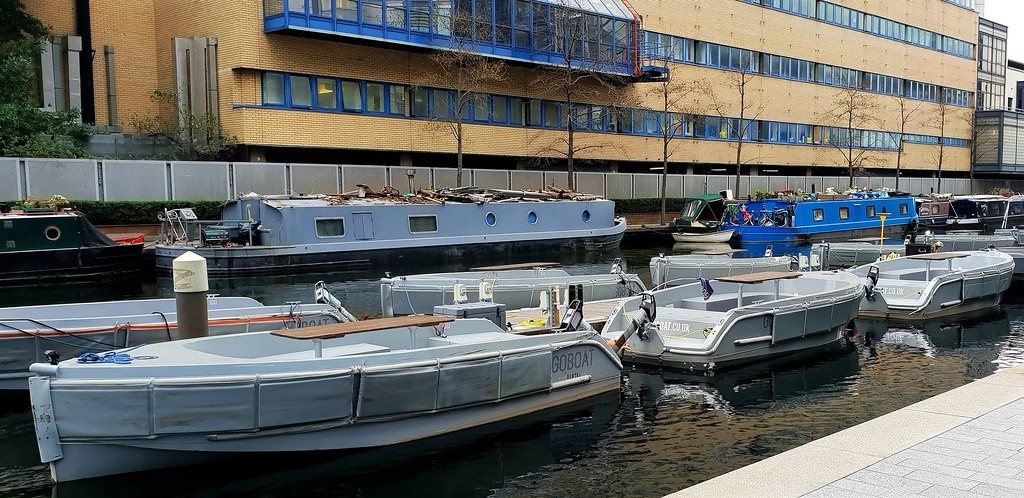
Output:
[672, 242, 733, 254]
[154, 190, 628, 267]
[671, 229, 736, 242]
[649, 190, 1024, 288]
[602, 271, 863, 372]
[0, 207, 145, 271]
[848, 244, 1016, 320]
[0, 277, 360, 391]
[380, 263, 647, 317]
[29, 314, 623, 484]
